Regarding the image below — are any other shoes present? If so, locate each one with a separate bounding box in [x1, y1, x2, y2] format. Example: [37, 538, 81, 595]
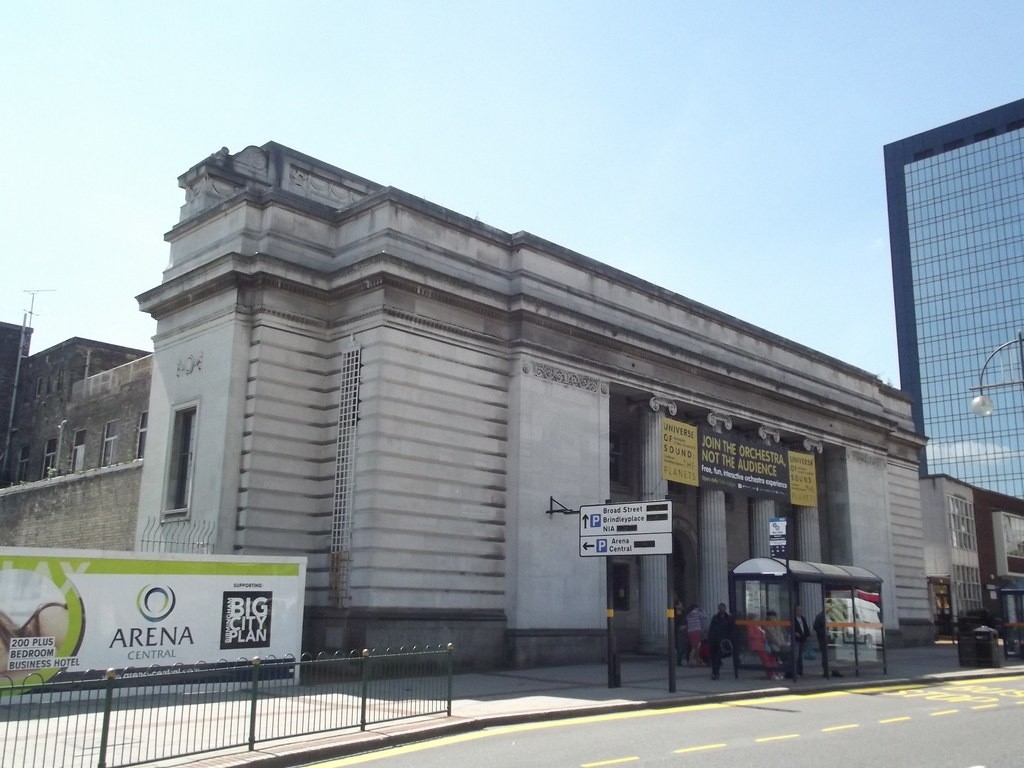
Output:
[785, 673, 793, 678]
[799, 674, 804, 677]
[677, 662, 684, 666]
[710, 672, 721, 680]
[689, 662, 707, 667]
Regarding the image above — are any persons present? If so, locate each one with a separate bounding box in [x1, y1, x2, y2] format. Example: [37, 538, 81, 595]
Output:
[813, 602, 844, 678]
[791, 604, 811, 678]
[747, 609, 801, 680]
[674, 601, 686, 666]
[707, 603, 733, 680]
[687, 604, 707, 667]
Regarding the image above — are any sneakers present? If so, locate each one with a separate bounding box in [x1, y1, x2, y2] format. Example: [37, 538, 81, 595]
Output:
[767, 672, 784, 681]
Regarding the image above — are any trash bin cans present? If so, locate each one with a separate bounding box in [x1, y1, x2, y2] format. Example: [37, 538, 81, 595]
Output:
[781, 642, 799, 679]
[973, 625, 1000, 668]
